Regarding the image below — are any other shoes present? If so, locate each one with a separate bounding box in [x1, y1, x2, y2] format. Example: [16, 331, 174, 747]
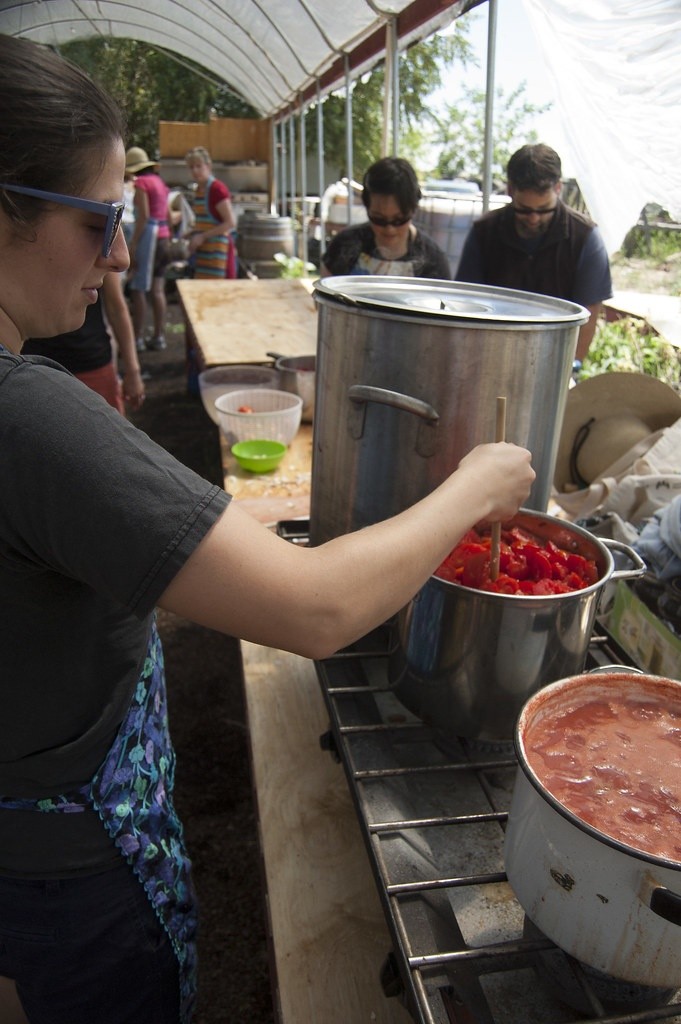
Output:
[137, 335, 167, 351]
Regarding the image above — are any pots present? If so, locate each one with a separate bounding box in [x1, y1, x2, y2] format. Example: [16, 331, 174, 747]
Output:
[388, 507, 646, 746]
[276, 355, 318, 423]
[502, 663, 681, 991]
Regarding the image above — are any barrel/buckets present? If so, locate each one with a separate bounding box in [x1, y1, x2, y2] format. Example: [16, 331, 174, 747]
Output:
[233, 212, 296, 279]
[310, 275, 591, 559]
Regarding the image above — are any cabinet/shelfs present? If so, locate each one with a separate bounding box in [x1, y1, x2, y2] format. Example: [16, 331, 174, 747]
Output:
[158, 157, 267, 215]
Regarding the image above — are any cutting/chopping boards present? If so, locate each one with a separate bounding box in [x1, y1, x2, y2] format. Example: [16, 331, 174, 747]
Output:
[176, 279, 317, 365]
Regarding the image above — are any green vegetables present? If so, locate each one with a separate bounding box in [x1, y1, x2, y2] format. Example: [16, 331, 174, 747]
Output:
[577, 313, 681, 397]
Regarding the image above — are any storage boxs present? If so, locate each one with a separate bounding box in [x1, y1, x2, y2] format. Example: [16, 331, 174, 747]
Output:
[607, 576, 681, 683]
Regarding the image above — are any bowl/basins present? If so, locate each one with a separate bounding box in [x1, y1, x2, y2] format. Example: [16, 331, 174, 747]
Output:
[199, 365, 284, 427]
[214, 389, 304, 450]
[231, 440, 286, 472]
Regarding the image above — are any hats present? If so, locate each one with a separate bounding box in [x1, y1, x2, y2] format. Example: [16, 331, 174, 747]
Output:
[553, 372, 681, 497]
[125, 146, 159, 173]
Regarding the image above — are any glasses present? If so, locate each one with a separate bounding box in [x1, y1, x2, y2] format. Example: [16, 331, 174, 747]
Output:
[512, 206, 557, 214]
[3, 183, 126, 258]
[368, 213, 414, 227]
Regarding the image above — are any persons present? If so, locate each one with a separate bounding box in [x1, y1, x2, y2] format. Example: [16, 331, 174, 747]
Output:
[116, 146, 171, 355]
[317, 156, 450, 279]
[181, 146, 236, 282]
[1, 36, 536, 1024]
[20, 217, 150, 410]
[453, 143, 613, 379]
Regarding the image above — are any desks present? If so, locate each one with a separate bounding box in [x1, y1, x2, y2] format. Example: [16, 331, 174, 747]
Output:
[219, 421, 681, 1024]
[175, 279, 319, 371]
